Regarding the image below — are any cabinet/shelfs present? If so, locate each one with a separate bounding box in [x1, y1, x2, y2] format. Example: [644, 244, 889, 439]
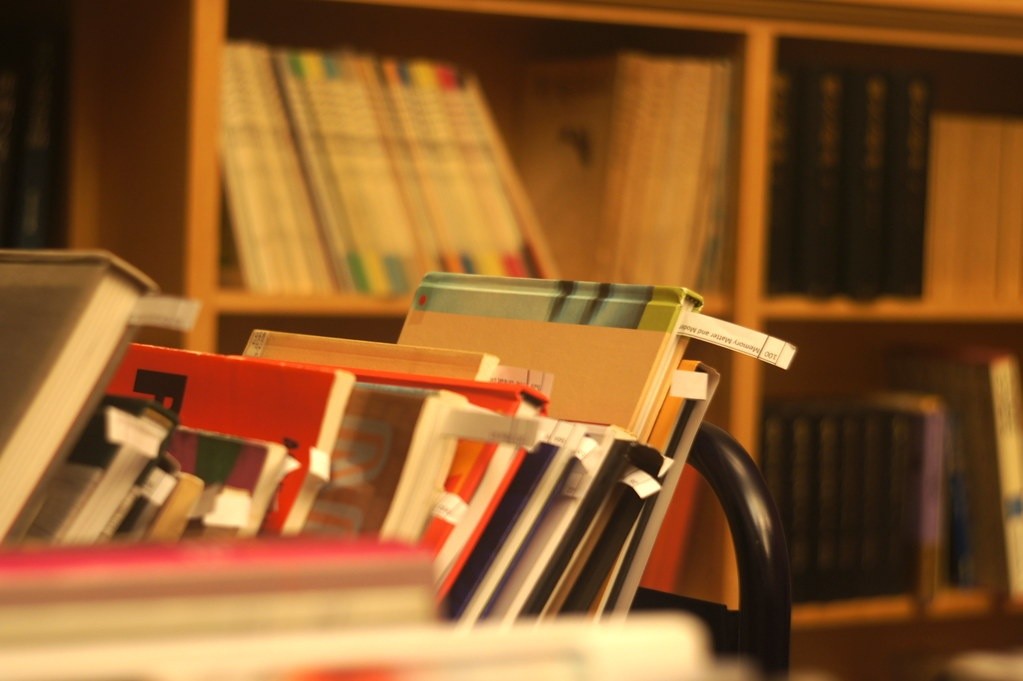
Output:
[63, 0, 1023, 681]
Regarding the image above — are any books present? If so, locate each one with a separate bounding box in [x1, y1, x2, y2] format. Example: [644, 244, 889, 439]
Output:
[0, 41, 1023, 681]
